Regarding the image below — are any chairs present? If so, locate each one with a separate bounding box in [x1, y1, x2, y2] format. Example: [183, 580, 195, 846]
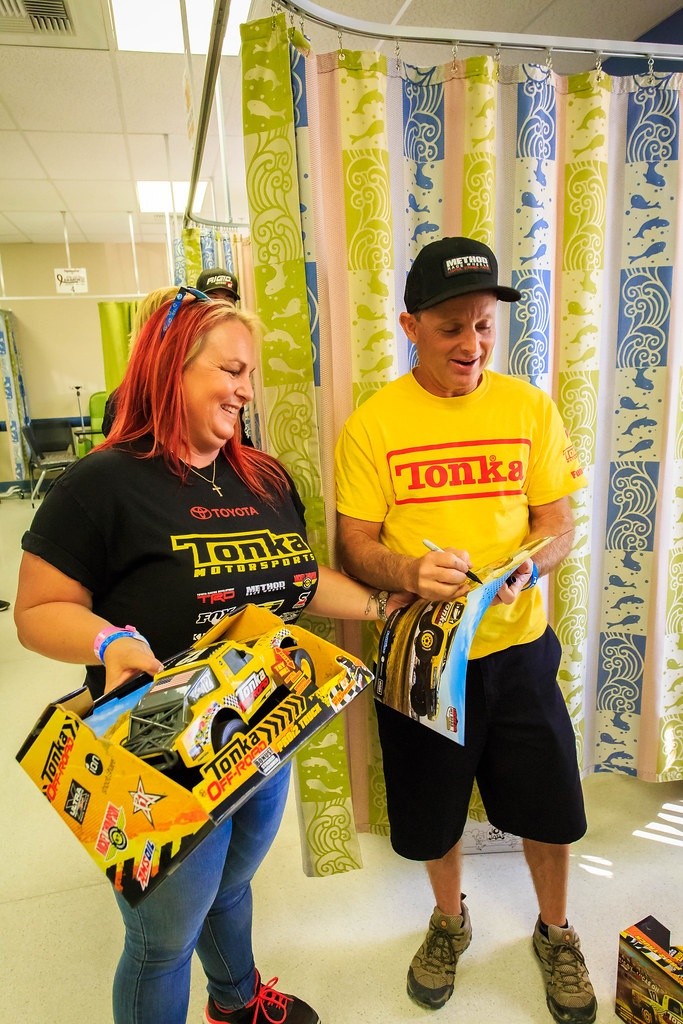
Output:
[22, 426, 80, 510]
[28, 420, 76, 499]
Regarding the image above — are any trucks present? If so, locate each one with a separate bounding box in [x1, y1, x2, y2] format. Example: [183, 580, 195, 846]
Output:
[632, 989, 683, 1024]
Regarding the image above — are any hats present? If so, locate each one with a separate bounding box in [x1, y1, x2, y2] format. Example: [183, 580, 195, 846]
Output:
[196, 267, 240, 302]
[404, 235, 521, 313]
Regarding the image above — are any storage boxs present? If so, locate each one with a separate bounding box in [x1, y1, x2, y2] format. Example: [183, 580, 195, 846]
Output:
[615, 915, 683, 1024]
[14, 603, 375, 909]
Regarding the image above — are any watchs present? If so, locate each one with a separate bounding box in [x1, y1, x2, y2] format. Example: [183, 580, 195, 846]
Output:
[378, 590, 400, 624]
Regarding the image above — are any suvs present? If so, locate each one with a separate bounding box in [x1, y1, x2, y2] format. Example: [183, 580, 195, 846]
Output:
[123, 625, 315, 792]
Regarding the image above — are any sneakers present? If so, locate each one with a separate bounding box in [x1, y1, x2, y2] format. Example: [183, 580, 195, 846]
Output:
[532, 912, 597, 1024]
[206, 968, 320, 1024]
[406, 891, 473, 1011]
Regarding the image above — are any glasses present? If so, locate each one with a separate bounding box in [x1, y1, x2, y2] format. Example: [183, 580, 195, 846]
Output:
[161, 286, 212, 343]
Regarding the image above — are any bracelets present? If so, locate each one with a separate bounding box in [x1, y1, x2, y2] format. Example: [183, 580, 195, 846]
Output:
[521, 559, 542, 596]
[92, 623, 153, 666]
[362, 588, 386, 620]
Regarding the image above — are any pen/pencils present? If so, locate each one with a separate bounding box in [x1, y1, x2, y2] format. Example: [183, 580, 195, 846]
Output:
[422, 539, 481, 584]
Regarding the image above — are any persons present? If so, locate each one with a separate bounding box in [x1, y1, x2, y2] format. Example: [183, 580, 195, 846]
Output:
[99, 266, 256, 452]
[13, 302, 418, 1023]
[329, 236, 600, 1024]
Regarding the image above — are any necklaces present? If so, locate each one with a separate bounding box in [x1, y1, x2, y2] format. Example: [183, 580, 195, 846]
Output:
[157, 437, 225, 502]
